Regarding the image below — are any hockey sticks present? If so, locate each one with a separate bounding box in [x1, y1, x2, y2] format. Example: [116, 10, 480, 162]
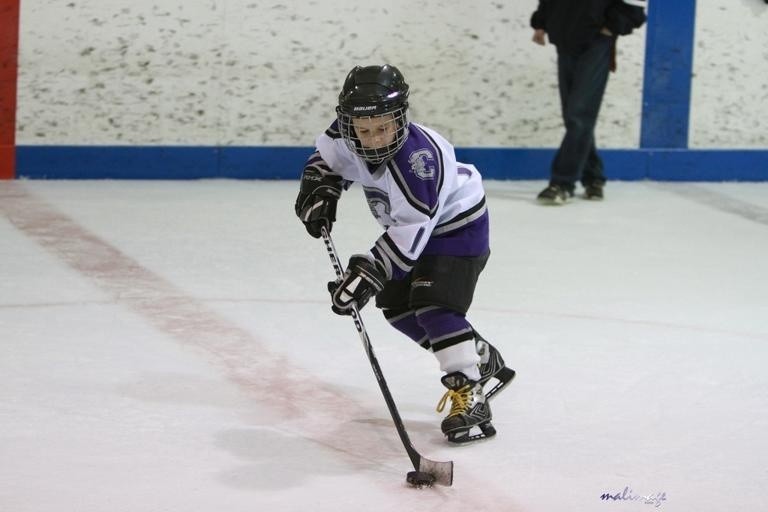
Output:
[318, 226, 453, 485]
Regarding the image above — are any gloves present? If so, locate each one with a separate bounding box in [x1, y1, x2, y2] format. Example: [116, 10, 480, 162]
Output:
[327, 254, 385, 315]
[295, 173, 342, 238]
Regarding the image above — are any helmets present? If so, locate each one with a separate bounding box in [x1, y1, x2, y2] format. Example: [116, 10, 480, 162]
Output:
[336, 65, 410, 165]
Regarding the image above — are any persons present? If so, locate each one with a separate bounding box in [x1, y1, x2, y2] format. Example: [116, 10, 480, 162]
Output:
[525, 0, 650, 206]
[292, 62, 507, 435]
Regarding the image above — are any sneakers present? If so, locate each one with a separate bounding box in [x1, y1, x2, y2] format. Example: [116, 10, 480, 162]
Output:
[586, 185, 603, 199]
[440, 327, 504, 431]
[538, 185, 574, 204]
